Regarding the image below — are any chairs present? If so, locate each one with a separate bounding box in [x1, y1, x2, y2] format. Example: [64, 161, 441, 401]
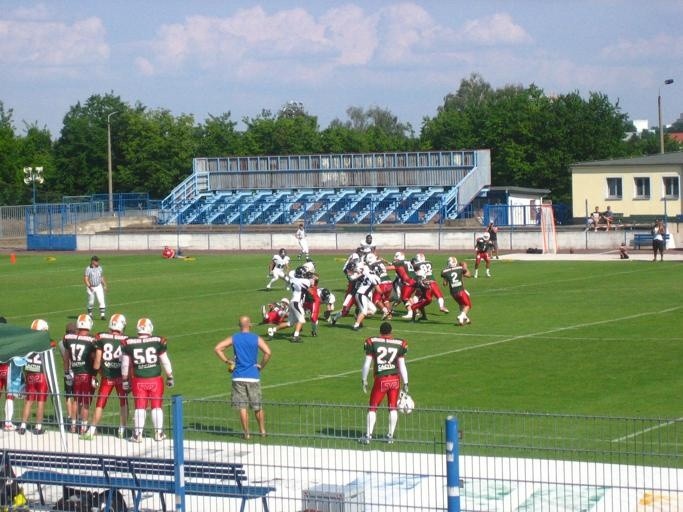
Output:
[155, 187, 461, 226]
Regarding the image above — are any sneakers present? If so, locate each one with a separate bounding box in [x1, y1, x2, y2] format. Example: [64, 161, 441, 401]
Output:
[266, 284, 271, 289]
[457, 314, 471, 326]
[5, 423, 45, 435]
[387, 433, 395, 445]
[243, 432, 267, 441]
[486, 272, 491, 277]
[324, 309, 337, 325]
[439, 306, 450, 313]
[403, 312, 428, 322]
[358, 434, 371, 445]
[89, 312, 107, 321]
[267, 326, 274, 341]
[70, 422, 94, 440]
[117, 428, 142, 443]
[297, 255, 309, 261]
[153, 430, 166, 441]
[290, 334, 303, 344]
[381, 310, 393, 321]
[474, 270, 479, 278]
[312, 329, 317, 336]
[351, 322, 359, 330]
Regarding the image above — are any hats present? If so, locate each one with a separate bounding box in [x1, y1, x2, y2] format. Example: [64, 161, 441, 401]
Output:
[90, 255, 102, 260]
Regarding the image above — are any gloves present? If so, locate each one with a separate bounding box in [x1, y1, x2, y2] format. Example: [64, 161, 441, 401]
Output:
[119, 380, 133, 392]
[165, 377, 176, 389]
[63, 374, 72, 387]
[363, 381, 369, 394]
[403, 383, 410, 394]
[90, 377, 99, 388]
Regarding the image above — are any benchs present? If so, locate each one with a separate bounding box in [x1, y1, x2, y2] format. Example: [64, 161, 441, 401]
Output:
[622, 213, 668, 230]
[0, 446, 276, 512]
[583, 211, 624, 231]
[628, 233, 670, 251]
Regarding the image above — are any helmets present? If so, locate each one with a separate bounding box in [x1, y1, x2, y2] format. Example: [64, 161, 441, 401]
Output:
[137, 317, 153, 336]
[483, 232, 490, 241]
[280, 297, 289, 307]
[346, 246, 377, 272]
[393, 251, 405, 261]
[29, 318, 48, 331]
[415, 253, 425, 262]
[295, 266, 307, 278]
[446, 256, 457, 268]
[279, 247, 286, 259]
[396, 389, 415, 414]
[320, 288, 330, 300]
[108, 313, 127, 334]
[75, 314, 94, 330]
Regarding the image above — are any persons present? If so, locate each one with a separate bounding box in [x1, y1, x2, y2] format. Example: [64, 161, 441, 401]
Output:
[582, 213, 594, 233]
[17, 318, 56, 436]
[473, 231, 491, 279]
[57, 323, 76, 420]
[121, 317, 175, 443]
[293, 223, 312, 262]
[590, 206, 601, 233]
[601, 205, 613, 232]
[161, 245, 186, 259]
[650, 218, 665, 261]
[259, 234, 472, 343]
[534, 207, 540, 225]
[61, 313, 99, 436]
[213, 315, 272, 439]
[76, 311, 129, 441]
[485, 219, 499, 259]
[0, 315, 18, 432]
[355, 322, 408, 445]
[82, 255, 108, 321]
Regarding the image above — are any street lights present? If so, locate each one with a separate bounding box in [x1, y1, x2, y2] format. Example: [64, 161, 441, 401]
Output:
[658, 77, 675, 153]
[105, 108, 125, 214]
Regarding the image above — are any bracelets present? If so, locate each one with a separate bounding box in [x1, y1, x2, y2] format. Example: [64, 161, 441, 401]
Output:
[225, 359, 229, 363]
[167, 256, 169, 258]
[88, 286, 91, 288]
